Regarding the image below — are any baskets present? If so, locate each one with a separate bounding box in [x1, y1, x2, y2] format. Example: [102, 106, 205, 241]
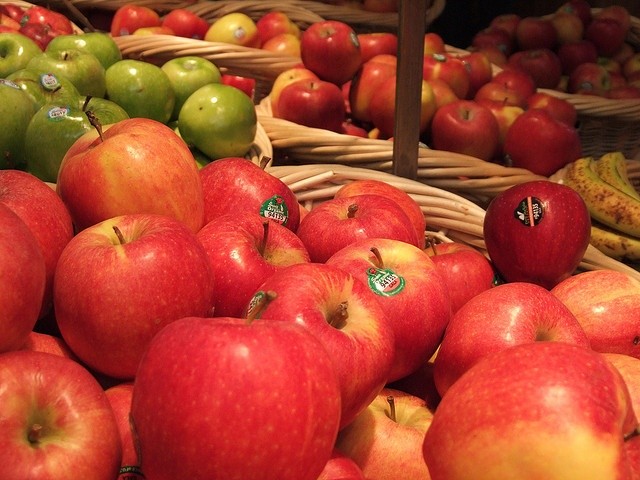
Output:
[255, 43, 548, 208]
[65, 1, 446, 37]
[466, 8, 640, 163]
[108, 2, 323, 103]
[266, 163, 639, 295]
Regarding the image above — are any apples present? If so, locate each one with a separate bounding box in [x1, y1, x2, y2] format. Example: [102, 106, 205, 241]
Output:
[111, 4, 302, 101]
[0, 33, 257, 182]
[433, 283, 590, 404]
[441, 48, 582, 177]
[597, 352, 640, 420]
[483, 180, 592, 291]
[271, 20, 444, 148]
[2, 117, 452, 480]
[420, 339, 640, 479]
[473, 3, 640, 99]
[550, 269, 640, 357]
[0, 2, 74, 52]
[425, 238, 495, 313]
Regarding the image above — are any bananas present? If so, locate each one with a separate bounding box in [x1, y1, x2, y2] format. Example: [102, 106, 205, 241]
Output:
[551, 151, 640, 263]
